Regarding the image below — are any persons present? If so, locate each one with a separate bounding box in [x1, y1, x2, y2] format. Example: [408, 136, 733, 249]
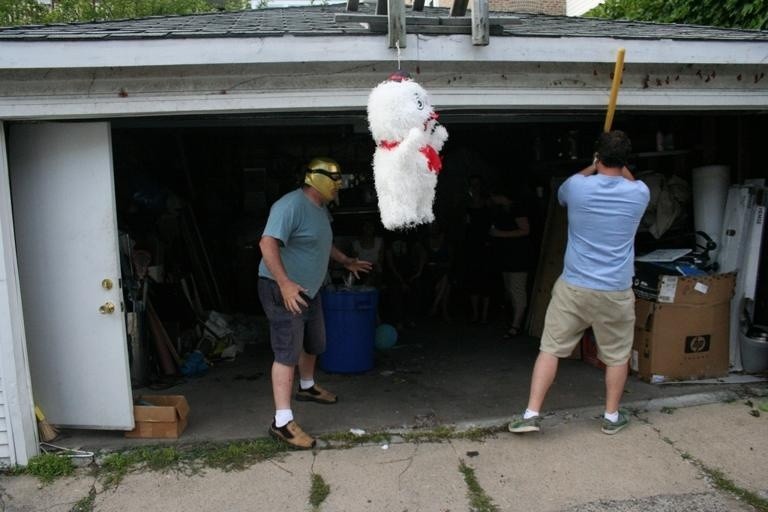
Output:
[505, 128, 650, 433]
[254, 156, 375, 448]
[338, 172, 536, 337]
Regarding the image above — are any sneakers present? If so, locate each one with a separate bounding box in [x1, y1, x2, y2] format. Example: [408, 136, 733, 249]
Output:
[508, 415, 543, 434]
[600, 407, 633, 435]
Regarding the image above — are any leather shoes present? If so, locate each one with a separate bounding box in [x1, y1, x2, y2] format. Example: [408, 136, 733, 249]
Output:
[294, 382, 338, 405]
[268, 415, 316, 450]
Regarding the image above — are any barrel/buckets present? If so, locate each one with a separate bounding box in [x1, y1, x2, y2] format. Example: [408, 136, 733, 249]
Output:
[319, 284, 375, 371]
[741, 330, 768, 376]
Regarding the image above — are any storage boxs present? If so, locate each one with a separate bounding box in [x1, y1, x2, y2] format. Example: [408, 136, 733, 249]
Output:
[126, 393, 192, 440]
[581, 271, 736, 384]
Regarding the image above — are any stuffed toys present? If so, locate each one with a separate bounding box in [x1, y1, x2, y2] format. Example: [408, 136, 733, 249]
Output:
[362, 75, 450, 232]
